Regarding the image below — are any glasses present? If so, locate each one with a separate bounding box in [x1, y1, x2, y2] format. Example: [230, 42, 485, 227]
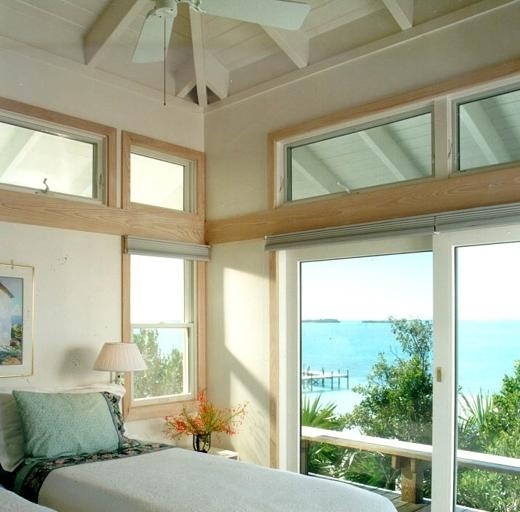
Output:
[92, 342, 148, 386]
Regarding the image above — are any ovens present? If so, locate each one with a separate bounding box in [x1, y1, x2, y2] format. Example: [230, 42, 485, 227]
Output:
[0, 436, 397, 512]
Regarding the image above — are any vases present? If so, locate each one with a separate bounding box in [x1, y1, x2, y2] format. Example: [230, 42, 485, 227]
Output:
[161, 388, 250, 448]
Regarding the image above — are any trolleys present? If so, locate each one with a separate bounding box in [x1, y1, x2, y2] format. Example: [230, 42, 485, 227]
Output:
[131, 1, 312, 65]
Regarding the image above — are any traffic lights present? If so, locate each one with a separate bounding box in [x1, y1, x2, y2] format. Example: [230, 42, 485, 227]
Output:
[0, 383, 140, 472]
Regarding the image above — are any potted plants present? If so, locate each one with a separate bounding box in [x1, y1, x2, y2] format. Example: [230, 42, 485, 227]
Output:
[0, 262, 36, 378]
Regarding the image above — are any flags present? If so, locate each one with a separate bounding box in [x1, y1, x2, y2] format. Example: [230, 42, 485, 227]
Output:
[192, 434, 211, 452]
